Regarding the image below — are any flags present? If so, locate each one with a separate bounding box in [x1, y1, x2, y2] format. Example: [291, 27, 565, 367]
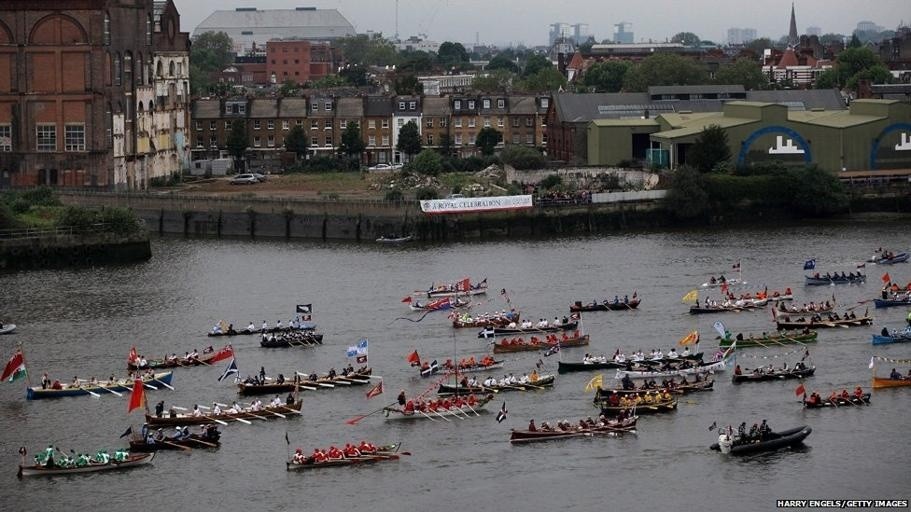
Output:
[0, 348, 25, 383]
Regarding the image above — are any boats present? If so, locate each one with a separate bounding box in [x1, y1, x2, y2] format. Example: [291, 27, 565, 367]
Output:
[375, 235, 413, 244]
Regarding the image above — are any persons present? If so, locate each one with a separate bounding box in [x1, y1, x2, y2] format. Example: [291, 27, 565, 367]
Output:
[33, 250, 911, 468]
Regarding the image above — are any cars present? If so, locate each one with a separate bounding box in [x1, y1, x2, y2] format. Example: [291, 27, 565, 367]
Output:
[368, 162, 406, 173]
[227, 172, 268, 185]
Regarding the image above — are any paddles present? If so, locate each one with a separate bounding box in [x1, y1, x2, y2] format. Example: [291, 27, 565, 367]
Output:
[570, 425, 638, 436]
[767, 373, 785, 380]
[327, 451, 411, 463]
[739, 306, 754, 312]
[419, 404, 480, 424]
[184, 435, 218, 448]
[153, 402, 301, 427]
[782, 368, 803, 380]
[813, 322, 861, 329]
[643, 405, 658, 410]
[262, 371, 384, 391]
[859, 397, 870, 407]
[156, 438, 193, 451]
[479, 382, 546, 393]
[81, 378, 176, 397]
[653, 403, 673, 408]
[728, 308, 739, 313]
